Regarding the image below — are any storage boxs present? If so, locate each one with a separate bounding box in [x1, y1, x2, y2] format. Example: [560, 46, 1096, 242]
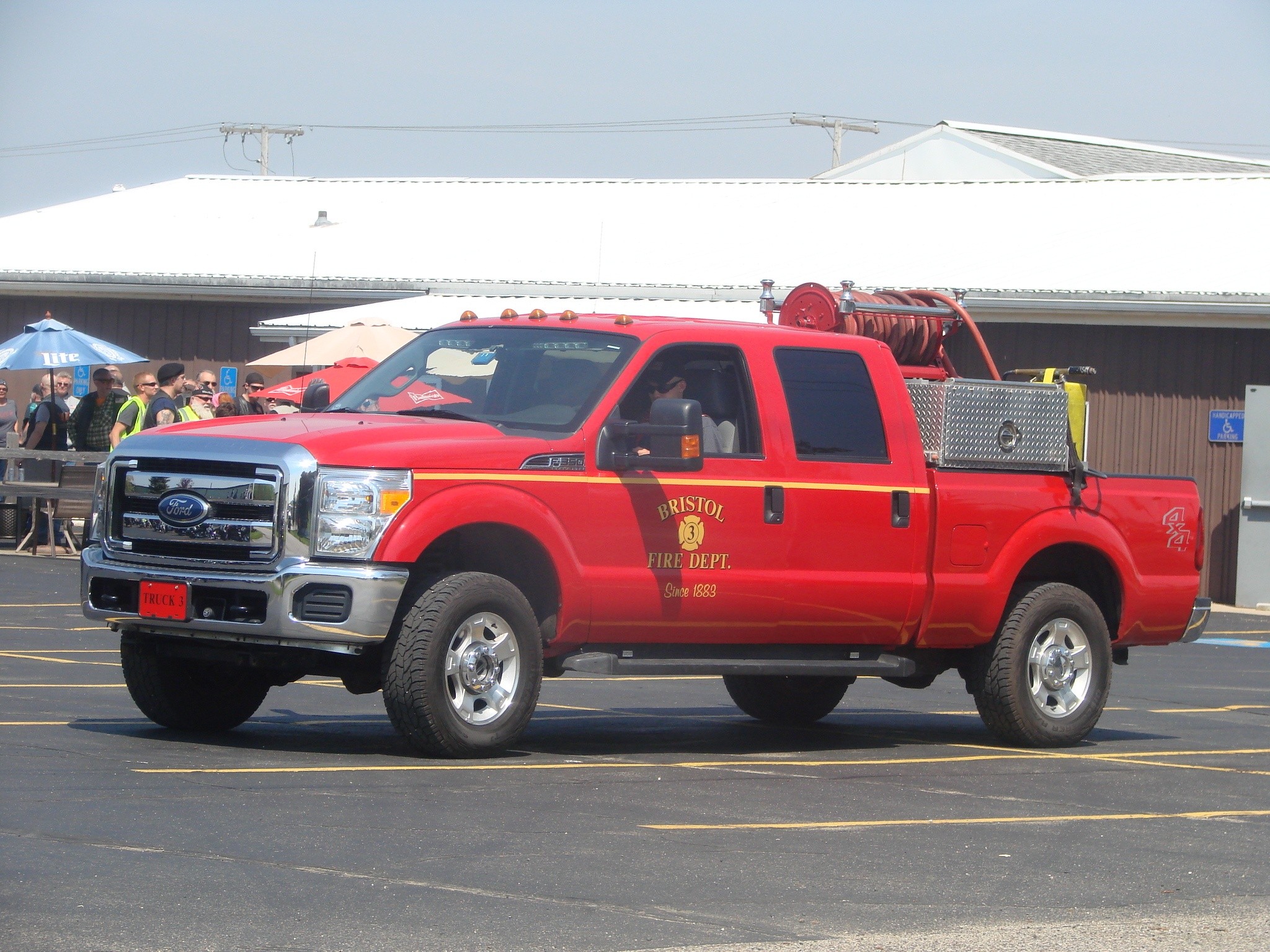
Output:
[904, 377, 1068, 472]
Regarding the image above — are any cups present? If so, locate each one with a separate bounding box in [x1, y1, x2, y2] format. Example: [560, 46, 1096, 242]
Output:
[8, 468, 14, 481]
[18, 468, 25, 482]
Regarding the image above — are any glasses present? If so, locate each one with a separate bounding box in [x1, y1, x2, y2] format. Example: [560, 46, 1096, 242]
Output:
[94, 378, 115, 385]
[645, 379, 683, 394]
[57, 382, 68, 387]
[249, 385, 264, 391]
[195, 396, 212, 402]
[204, 381, 217, 387]
[0, 388, 6, 391]
[136, 382, 156, 387]
[267, 398, 275, 402]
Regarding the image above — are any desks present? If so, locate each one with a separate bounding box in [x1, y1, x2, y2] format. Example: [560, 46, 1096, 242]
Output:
[3, 479, 77, 558]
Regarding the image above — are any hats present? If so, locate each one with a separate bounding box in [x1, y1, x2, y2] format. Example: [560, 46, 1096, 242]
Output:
[191, 384, 212, 396]
[158, 363, 184, 382]
[32, 384, 43, 397]
[242, 372, 264, 387]
[646, 357, 686, 384]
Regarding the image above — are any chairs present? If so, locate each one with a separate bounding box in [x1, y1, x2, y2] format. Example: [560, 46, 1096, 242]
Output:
[683, 369, 741, 454]
[533, 359, 603, 409]
[29, 465, 97, 555]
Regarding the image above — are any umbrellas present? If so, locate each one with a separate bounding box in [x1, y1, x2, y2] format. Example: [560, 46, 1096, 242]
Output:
[0, 311, 149, 507]
[244, 317, 498, 412]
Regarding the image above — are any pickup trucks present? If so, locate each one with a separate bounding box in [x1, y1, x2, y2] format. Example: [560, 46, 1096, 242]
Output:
[78, 280, 1212, 760]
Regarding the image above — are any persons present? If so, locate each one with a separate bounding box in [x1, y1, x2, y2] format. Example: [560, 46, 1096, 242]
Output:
[15, 364, 298, 550]
[632, 363, 723, 456]
[0, 380, 18, 502]
